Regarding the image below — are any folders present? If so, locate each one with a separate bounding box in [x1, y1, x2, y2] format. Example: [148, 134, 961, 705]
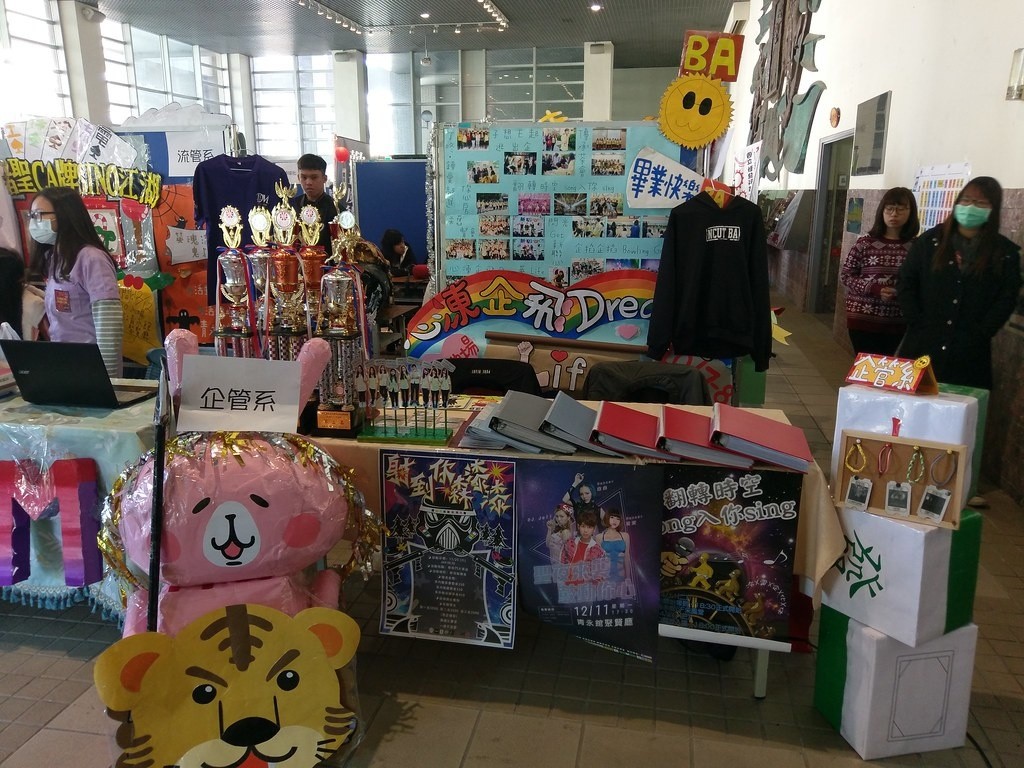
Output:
[588, 400, 683, 463]
[654, 405, 757, 472]
[464, 401, 545, 454]
[537, 391, 628, 459]
[488, 390, 583, 456]
[708, 402, 814, 475]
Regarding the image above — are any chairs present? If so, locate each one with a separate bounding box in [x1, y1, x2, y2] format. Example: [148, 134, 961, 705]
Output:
[584, 363, 713, 406]
[433, 359, 542, 398]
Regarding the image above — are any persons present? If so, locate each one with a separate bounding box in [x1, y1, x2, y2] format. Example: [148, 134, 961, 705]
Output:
[592, 159, 624, 176]
[543, 153, 575, 175]
[544, 472, 632, 589]
[505, 155, 536, 175]
[381, 228, 416, 277]
[284, 154, 345, 258]
[900, 176, 1022, 507]
[596, 137, 622, 149]
[0, 187, 124, 377]
[356, 365, 451, 409]
[445, 191, 667, 289]
[469, 166, 497, 183]
[543, 128, 570, 152]
[458, 129, 489, 150]
[840, 187, 920, 358]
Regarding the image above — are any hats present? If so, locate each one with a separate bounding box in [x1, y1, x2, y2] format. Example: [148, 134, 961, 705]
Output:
[554, 503, 575, 523]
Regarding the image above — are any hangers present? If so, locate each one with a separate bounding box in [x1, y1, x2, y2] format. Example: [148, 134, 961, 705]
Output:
[670, 177, 760, 214]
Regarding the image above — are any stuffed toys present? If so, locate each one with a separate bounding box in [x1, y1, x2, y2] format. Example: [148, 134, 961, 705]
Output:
[101, 331, 351, 639]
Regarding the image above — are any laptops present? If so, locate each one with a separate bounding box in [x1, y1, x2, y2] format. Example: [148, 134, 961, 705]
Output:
[0, 339, 159, 410]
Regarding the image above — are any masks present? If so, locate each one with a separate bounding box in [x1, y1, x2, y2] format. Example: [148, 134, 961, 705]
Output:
[29, 218, 57, 245]
[953, 204, 992, 228]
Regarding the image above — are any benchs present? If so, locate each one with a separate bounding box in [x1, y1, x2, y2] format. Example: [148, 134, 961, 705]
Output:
[377, 284, 425, 352]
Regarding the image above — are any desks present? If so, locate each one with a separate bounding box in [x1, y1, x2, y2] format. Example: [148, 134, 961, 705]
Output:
[0, 376, 848, 703]
[381, 305, 419, 356]
[390, 275, 430, 295]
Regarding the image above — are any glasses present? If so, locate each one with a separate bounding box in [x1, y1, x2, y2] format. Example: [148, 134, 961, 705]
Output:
[883, 207, 910, 215]
[957, 198, 990, 208]
[23, 210, 56, 223]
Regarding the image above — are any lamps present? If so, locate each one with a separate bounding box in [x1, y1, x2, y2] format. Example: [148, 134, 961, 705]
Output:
[298, 0, 510, 35]
[420, 35, 432, 66]
[421, 110, 433, 129]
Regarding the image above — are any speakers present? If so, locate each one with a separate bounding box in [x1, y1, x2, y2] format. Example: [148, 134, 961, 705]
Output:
[590, 44, 604, 54]
[334, 52, 350, 62]
[83, 6, 106, 23]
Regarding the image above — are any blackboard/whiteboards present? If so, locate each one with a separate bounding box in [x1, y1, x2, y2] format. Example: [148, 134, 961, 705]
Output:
[438, 120, 705, 359]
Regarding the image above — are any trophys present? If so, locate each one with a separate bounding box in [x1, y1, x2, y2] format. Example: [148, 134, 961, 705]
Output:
[213, 204, 359, 330]
